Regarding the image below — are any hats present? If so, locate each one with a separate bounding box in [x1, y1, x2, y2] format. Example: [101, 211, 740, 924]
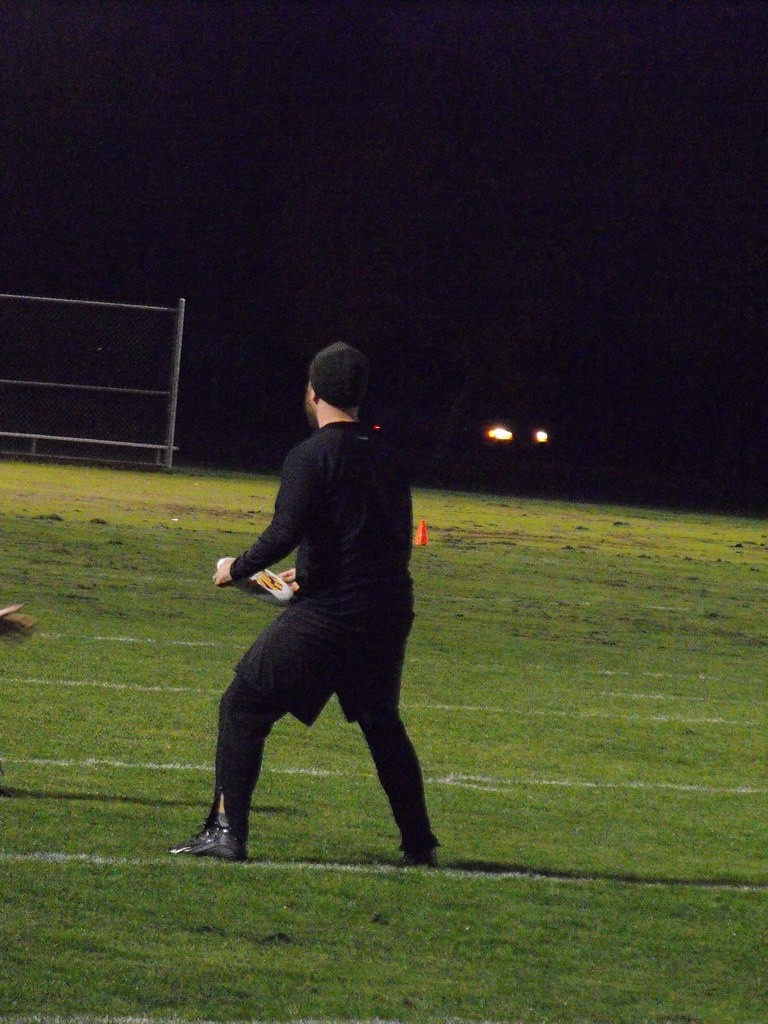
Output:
[310, 341, 370, 408]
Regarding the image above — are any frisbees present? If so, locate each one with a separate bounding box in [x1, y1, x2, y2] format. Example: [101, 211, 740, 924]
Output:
[209, 552, 297, 612]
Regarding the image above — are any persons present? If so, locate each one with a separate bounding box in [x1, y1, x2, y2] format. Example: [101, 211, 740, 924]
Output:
[1, 603, 38, 644]
[165, 341, 440, 865]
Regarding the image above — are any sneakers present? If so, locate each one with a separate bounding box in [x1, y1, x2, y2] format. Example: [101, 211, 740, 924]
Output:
[392, 848, 437, 867]
[168, 823, 245, 860]
